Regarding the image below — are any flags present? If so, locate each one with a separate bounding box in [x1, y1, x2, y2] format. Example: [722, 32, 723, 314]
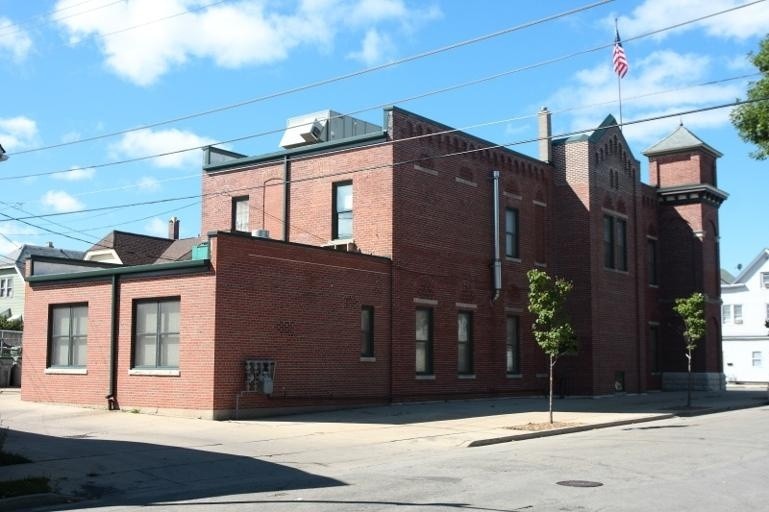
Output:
[613, 29, 628, 81]
